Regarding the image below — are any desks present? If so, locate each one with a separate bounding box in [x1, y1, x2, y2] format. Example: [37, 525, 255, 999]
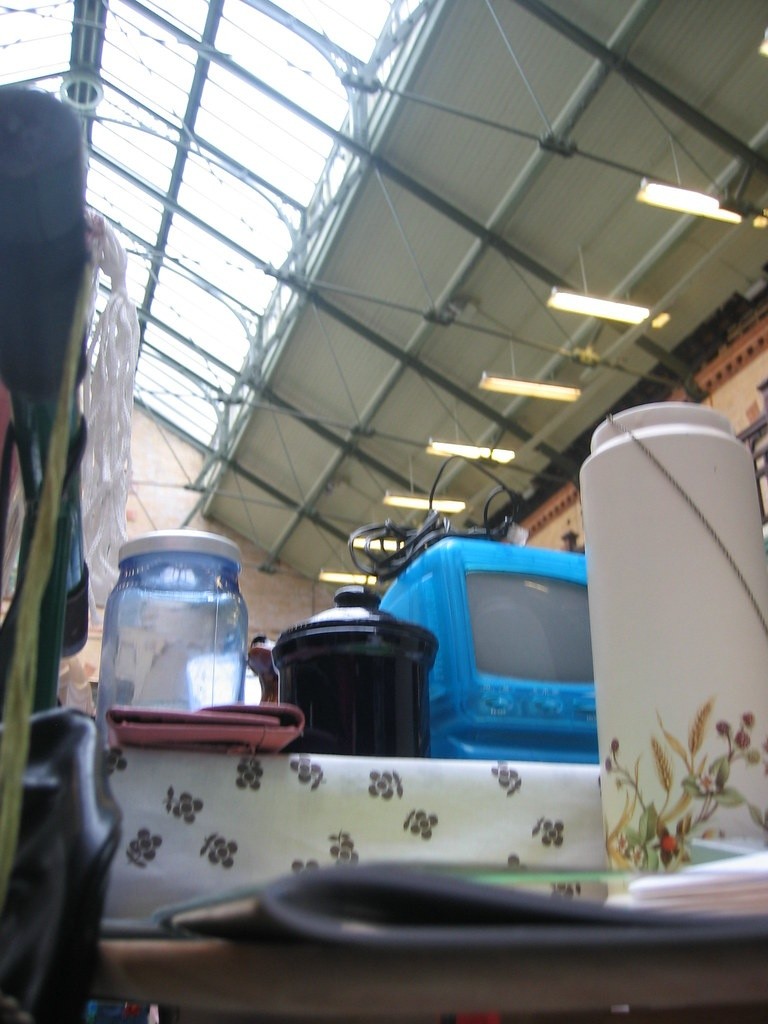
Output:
[104, 745, 610, 921]
[94, 936, 768, 1024]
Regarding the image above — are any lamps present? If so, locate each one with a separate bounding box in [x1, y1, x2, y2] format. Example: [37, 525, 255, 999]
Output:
[425, 401, 515, 464]
[318, 545, 378, 585]
[548, 244, 650, 325]
[636, 137, 742, 224]
[381, 457, 467, 515]
[353, 512, 403, 553]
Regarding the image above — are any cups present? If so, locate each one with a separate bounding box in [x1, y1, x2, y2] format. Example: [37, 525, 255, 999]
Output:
[271, 586, 439, 759]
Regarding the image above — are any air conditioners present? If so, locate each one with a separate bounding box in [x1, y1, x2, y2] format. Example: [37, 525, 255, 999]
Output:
[477, 338, 583, 402]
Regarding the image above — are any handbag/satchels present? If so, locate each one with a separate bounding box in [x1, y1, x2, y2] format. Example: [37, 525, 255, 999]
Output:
[108, 706, 304, 754]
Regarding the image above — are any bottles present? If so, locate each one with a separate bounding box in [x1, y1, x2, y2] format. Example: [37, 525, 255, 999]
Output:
[91, 530, 248, 817]
[580, 402, 768, 899]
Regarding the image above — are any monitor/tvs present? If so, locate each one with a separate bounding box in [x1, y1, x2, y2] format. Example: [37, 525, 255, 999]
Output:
[377, 536, 600, 766]
